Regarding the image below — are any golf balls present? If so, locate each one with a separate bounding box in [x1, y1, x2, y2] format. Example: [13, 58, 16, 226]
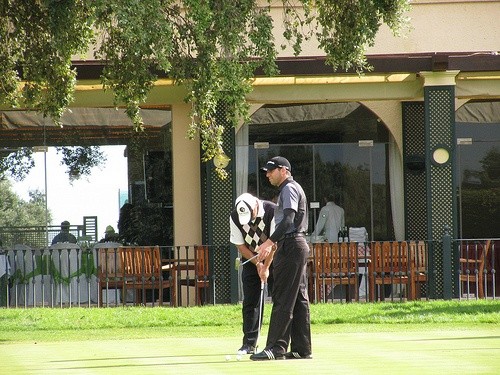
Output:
[225, 355, 231, 361]
[236, 350, 247, 356]
[236, 356, 243, 362]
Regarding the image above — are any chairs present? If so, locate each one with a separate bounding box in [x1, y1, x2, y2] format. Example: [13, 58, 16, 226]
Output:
[98, 244, 208, 306]
[460, 240, 491, 299]
[306, 240, 428, 302]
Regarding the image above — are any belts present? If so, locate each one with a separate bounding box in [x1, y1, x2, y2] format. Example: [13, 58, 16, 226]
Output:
[279, 232, 305, 241]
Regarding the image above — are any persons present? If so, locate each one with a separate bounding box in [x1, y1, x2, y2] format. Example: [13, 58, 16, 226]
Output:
[249, 155, 312, 361]
[230, 193, 290, 354]
[52, 221, 76, 245]
[104, 225, 116, 241]
[312, 188, 345, 242]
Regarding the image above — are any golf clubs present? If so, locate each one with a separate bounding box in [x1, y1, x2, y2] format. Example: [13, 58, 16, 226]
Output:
[255, 279, 265, 354]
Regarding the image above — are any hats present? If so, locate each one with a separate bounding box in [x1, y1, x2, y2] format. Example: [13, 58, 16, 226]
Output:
[260, 156, 292, 171]
[236, 192, 256, 225]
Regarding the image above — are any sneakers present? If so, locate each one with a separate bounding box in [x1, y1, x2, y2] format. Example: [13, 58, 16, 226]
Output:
[238, 344, 255, 355]
[251, 348, 286, 360]
[285, 351, 312, 359]
